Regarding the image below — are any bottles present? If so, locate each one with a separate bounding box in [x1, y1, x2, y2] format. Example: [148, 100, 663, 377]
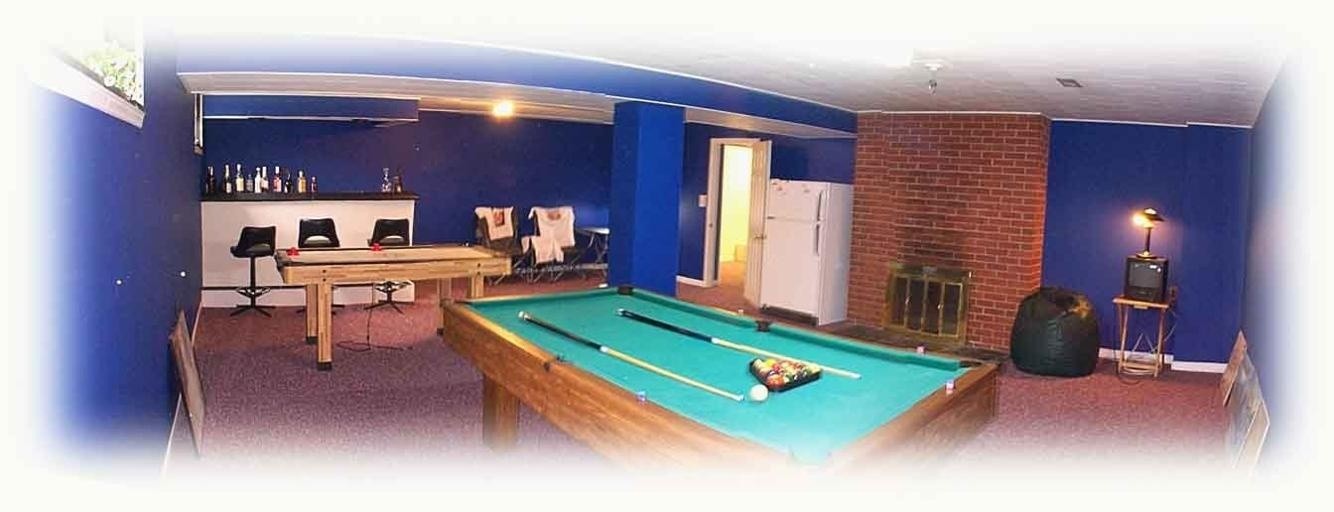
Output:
[203, 164, 318, 196]
[383, 166, 402, 196]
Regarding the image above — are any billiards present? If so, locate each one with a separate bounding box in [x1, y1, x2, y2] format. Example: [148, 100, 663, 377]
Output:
[751, 357, 819, 386]
[749, 384, 770, 400]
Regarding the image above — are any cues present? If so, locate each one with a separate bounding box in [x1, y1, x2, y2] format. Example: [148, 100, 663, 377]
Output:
[619, 307, 861, 380]
[516, 310, 742, 405]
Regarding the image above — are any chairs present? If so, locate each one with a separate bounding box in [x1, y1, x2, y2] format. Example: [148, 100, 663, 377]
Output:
[363, 218, 415, 315]
[530, 207, 588, 282]
[296, 218, 345, 315]
[475, 206, 534, 285]
[230, 225, 276, 318]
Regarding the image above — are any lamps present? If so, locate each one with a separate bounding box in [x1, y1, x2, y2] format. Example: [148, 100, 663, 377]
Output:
[1133, 207, 1165, 259]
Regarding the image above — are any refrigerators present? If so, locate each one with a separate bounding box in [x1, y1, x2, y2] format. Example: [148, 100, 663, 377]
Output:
[759, 178, 854, 328]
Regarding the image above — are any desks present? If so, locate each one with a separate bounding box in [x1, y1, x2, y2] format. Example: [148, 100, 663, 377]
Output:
[1113, 293, 1170, 377]
[586, 227, 610, 277]
[274, 243, 512, 370]
[441, 284, 998, 476]
